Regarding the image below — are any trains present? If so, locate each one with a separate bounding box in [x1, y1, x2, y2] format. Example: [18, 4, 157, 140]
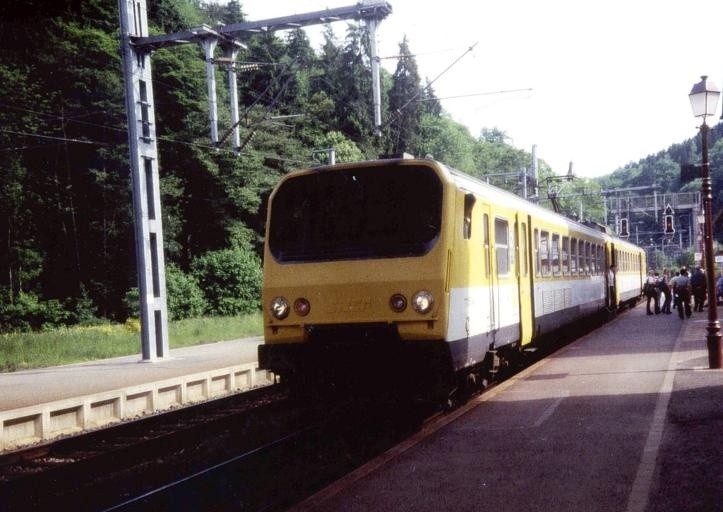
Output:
[253, 149, 649, 410]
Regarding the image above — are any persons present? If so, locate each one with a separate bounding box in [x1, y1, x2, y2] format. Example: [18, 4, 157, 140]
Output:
[606, 262, 617, 309]
[638, 264, 723, 321]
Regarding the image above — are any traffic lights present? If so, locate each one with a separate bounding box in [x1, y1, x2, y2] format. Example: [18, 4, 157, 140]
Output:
[665, 215, 676, 234]
[618, 217, 631, 237]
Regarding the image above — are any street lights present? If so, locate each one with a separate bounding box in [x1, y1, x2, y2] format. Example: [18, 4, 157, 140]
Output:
[688, 75, 722, 367]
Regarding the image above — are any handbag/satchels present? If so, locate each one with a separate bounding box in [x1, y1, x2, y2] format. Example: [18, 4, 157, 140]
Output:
[659, 282, 669, 294]
[643, 283, 656, 297]
